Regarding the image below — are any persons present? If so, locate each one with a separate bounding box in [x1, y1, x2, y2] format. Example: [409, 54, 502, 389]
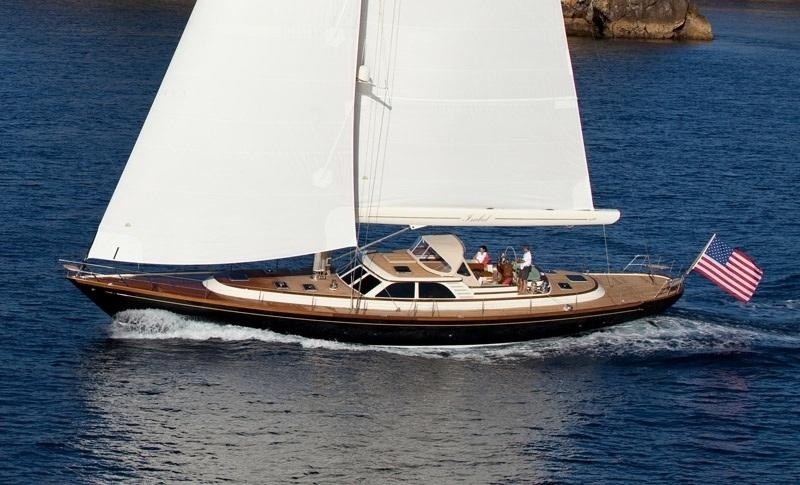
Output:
[515, 242, 532, 295]
[471, 244, 490, 268]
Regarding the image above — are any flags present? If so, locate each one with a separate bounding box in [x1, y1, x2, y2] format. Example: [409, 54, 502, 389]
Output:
[693, 233, 764, 304]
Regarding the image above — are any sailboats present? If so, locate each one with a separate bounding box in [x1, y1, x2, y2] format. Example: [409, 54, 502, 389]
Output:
[56, 0, 686, 349]
